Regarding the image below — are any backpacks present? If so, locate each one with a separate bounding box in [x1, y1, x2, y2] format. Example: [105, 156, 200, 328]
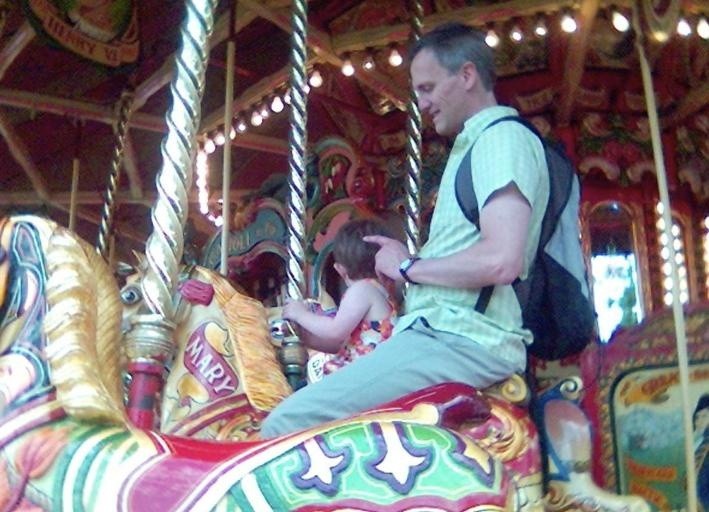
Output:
[455, 115, 595, 361]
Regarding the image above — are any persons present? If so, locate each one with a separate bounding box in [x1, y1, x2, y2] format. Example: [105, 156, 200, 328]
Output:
[256, 21, 554, 443]
[282, 221, 397, 377]
